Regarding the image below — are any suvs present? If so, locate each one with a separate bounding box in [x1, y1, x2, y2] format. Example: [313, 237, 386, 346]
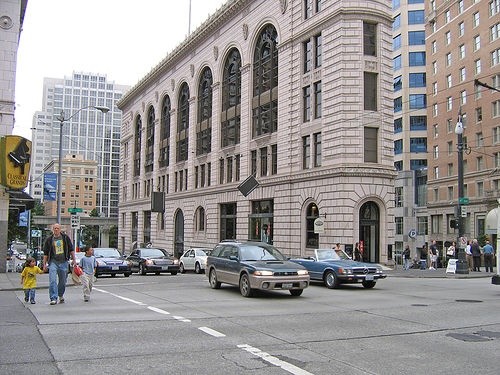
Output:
[203, 239, 311, 297]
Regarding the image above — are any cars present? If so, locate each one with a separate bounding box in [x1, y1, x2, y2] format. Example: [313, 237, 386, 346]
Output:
[69, 251, 85, 273]
[178, 247, 214, 274]
[90, 247, 133, 278]
[126, 248, 181, 276]
[16, 262, 24, 272]
[289, 247, 389, 289]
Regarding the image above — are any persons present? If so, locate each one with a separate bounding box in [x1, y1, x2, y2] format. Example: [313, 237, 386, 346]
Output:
[482, 241, 494, 273]
[402, 245, 411, 270]
[471, 239, 482, 271]
[336, 242, 342, 249]
[448, 242, 455, 258]
[79, 247, 96, 302]
[21, 259, 44, 303]
[354, 243, 362, 261]
[465, 240, 473, 270]
[44, 224, 79, 304]
[429, 240, 439, 271]
[420, 245, 428, 270]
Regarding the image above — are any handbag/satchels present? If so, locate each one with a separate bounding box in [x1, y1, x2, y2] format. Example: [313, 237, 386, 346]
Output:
[446, 246, 453, 256]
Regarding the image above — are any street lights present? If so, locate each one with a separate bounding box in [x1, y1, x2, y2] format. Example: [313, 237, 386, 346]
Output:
[453, 112, 470, 274]
[56, 104, 111, 225]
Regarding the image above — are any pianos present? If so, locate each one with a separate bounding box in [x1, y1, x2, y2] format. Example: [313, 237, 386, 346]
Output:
[8, 138, 30, 174]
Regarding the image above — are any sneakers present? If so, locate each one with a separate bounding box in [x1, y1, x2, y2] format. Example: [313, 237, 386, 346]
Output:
[84, 296, 88, 302]
[30, 299, 35, 304]
[25, 297, 29, 302]
[50, 301, 57, 305]
[59, 296, 65, 301]
